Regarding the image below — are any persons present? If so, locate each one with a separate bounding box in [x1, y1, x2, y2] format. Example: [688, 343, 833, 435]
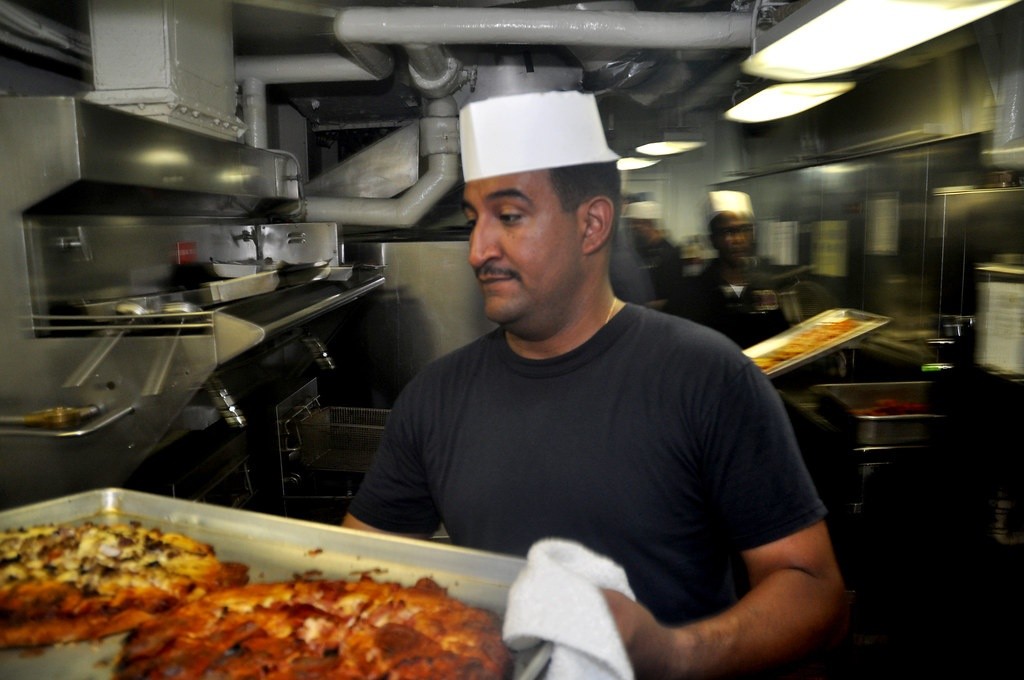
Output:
[625, 203, 685, 314]
[689, 207, 793, 351]
[339, 91, 840, 680]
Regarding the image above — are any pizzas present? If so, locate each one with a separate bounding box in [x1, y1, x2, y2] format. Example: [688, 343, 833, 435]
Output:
[750, 318, 856, 369]
[0, 524, 250, 648]
[110, 577, 516, 680]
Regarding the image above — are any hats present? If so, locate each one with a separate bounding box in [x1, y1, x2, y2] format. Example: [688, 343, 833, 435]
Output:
[620, 198, 657, 220]
[460, 90, 621, 183]
